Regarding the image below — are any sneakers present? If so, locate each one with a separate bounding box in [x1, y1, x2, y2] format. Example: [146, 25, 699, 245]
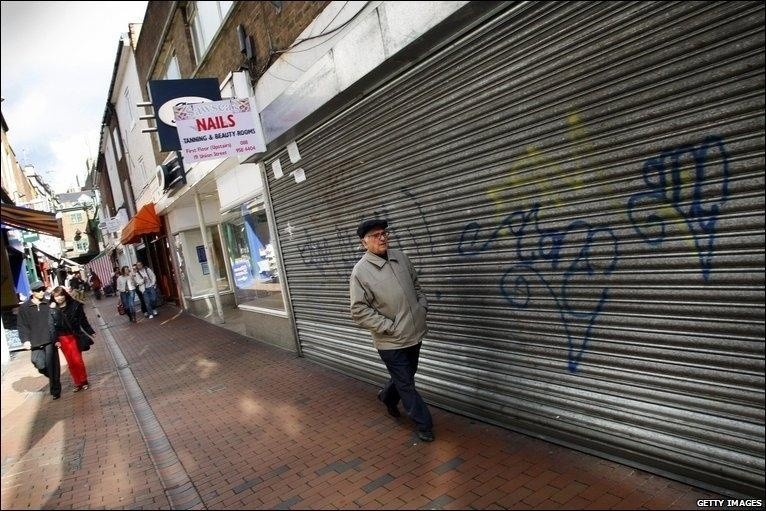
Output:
[152, 310, 158, 315]
[148, 314, 154, 319]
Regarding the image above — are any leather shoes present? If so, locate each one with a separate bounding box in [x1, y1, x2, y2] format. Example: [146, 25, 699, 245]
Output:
[82, 385, 88, 390]
[376, 389, 401, 418]
[412, 422, 435, 442]
[53, 392, 60, 399]
[73, 385, 80, 392]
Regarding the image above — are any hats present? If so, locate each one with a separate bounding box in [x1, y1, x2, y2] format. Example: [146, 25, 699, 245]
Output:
[29, 280, 47, 291]
[357, 218, 388, 239]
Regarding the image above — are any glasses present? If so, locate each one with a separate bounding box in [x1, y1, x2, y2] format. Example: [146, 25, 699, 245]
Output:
[371, 232, 388, 241]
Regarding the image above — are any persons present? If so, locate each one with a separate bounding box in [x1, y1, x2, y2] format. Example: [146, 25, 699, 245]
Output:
[17, 281, 61, 401]
[46, 288, 97, 391]
[63, 260, 159, 324]
[347, 218, 438, 441]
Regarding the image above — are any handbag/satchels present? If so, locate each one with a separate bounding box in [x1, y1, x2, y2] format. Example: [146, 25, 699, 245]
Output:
[116, 301, 126, 315]
